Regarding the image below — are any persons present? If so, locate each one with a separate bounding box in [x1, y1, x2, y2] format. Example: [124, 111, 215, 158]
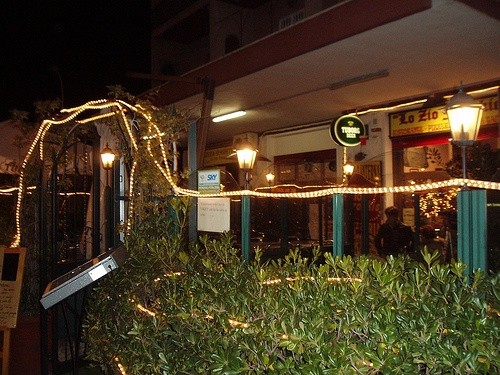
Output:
[419, 225, 446, 269]
[375, 206, 419, 264]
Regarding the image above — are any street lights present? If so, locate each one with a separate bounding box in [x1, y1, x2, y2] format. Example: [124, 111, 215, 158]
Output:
[233, 143, 258, 264]
[101, 142, 115, 250]
[446, 89, 485, 285]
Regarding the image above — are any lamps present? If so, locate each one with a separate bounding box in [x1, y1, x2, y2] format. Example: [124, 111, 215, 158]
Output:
[211, 109, 247, 123]
[330, 69, 390, 91]
[439, 81, 486, 191]
[342, 158, 354, 187]
[232, 134, 259, 184]
[265, 169, 275, 193]
[99, 142, 117, 188]
